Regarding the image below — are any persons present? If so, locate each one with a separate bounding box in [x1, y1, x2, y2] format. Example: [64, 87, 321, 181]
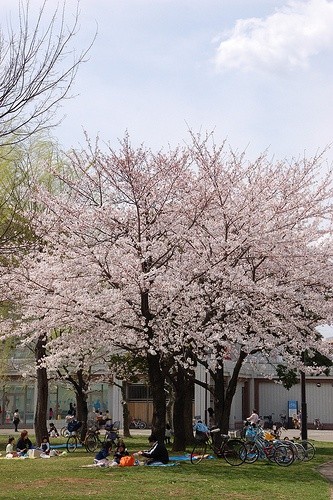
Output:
[13, 409, 20, 432]
[6, 412, 11, 423]
[165, 423, 172, 444]
[48, 423, 59, 436]
[138, 434, 169, 465]
[49, 408, 53, 420]
[192, 407, 215, 444]
[67, 403, 76, 416]
[247, 409, 259, 428]
[93, 443, 131, 467]
[6, 430, 32, 457]
[296, 410, 302, 430]
[39, 437, 50, 455]
[87, 408, 112, 427]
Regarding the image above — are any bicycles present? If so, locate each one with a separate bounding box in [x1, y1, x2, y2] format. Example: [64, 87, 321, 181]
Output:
[128, 418, 146, 430]
[59, 417, 119, 454]
[189, 421, 248, 466]
[241, 411, 322, 467]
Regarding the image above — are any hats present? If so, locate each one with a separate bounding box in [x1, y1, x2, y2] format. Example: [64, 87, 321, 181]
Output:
[49, 423, 54, 427]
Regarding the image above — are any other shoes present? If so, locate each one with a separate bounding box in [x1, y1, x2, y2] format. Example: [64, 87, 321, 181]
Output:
[15, 431, 18, 432]
